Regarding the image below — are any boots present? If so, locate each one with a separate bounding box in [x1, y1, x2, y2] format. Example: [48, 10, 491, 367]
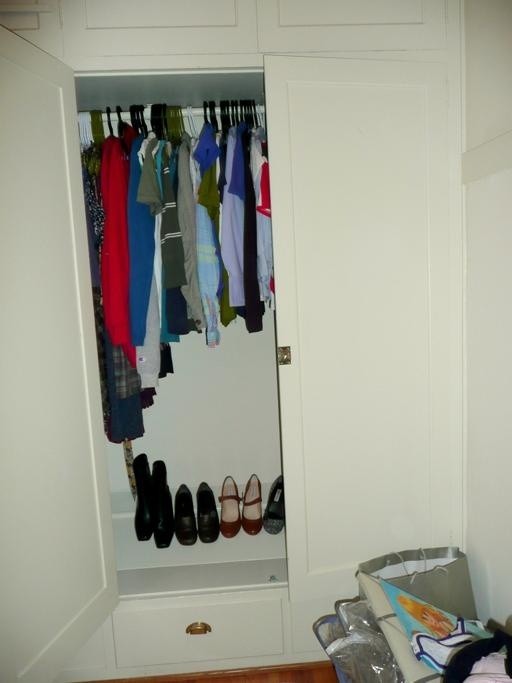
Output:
[133, 453, 154, 542]
[149, 460, 174, 548]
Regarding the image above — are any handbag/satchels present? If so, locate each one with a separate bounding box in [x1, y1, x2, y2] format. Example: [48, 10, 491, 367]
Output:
[359, 547, 477, 627]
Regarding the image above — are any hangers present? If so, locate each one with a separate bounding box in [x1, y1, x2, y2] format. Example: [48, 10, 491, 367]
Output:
[75, 97, 267, 151]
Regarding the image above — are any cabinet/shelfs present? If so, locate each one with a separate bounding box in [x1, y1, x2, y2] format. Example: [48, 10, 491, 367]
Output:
[0, 0, 466, 683]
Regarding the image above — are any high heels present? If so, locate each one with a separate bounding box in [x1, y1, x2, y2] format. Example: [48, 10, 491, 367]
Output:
[219, 476, 242, 539]
[262, 475, 285, 535]
[242, 474, 263, 535]
[175, 483, 198, 545]
[196, 481, 219, 544]
